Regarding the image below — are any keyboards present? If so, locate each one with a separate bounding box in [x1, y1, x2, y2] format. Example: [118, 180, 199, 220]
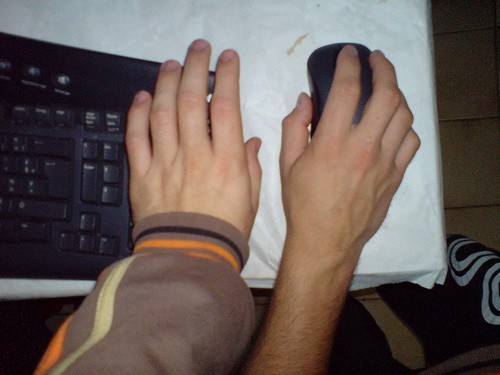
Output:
[0, 33, 216, 281]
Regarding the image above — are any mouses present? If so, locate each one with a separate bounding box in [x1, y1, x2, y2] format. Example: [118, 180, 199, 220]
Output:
[307, 43, 374, 140]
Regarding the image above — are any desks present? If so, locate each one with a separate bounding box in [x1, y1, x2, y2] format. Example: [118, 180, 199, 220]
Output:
[0, 0, 451, 300]
